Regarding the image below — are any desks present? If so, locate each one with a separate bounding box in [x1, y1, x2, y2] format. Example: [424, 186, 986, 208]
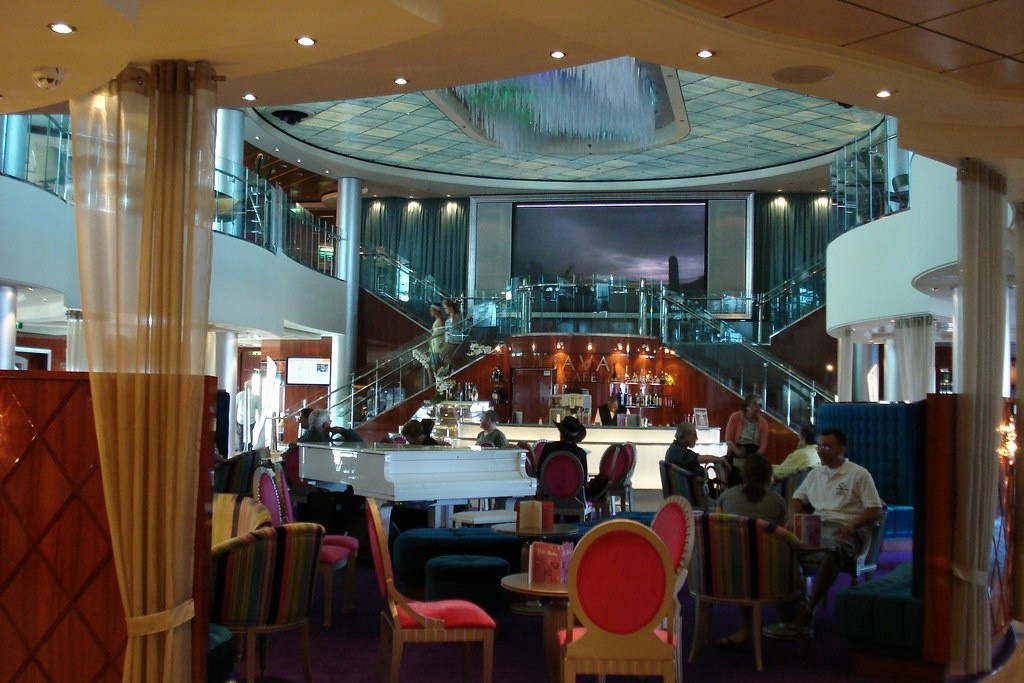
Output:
[489, 520, 579, 617]
[762, 542, 835, 640]
[500, 573, 575, 683]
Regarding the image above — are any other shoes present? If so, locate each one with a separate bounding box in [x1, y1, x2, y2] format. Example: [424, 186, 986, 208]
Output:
[780, 592, 810, 621]
[713, 632, 752, 645]
[762, 623, 815, 640]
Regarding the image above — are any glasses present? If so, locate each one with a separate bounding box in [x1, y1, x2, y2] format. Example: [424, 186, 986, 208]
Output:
[816, 443, 839, 451]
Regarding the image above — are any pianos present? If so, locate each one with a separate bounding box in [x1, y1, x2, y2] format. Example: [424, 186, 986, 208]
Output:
[296, 441, 537, 544]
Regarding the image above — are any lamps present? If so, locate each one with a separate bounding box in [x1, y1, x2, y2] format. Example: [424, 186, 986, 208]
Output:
[557, 338, 565, 349]
[587, 339, 593, 351]
[616, 339, 623, 352]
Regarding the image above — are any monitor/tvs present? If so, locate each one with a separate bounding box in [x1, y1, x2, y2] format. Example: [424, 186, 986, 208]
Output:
[286, 357, 331, 386]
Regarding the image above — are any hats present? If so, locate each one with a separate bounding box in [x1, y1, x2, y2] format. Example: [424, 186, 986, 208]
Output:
[552, 416, 587, 443]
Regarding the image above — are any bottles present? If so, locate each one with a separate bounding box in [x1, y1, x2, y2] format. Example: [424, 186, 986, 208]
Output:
[472, 386, 478, 401]
[612, 364, 672, 383]
[617, 387, 662, 407]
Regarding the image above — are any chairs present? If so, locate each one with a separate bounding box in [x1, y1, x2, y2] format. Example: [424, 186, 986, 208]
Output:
[592, 442, 636, 515]
[517, 440, 536, 474]
[534, 440, 550, 470]
[283, 445, 307, 504]
[366, 497, 496, 683]
[208, 523, 325, 683]
[557, 519, 679, 683]
[648, 494, 696, 683]
[773, 466, 815, 511]
[590, 445, 631, 514]
[687, 514, 806, 674]
[32, 146, 66, 189]
[214, 197, 241, 234]
[211, 493, 273, 547]
[804, 499, 887, 612]
[538, 452, 592, 524]
[252, 468, 352, 632]
[274, 463, 360, 612]
[658, 460, 704, 509]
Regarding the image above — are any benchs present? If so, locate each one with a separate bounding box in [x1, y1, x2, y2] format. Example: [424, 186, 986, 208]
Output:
[392, 527, 589, 602]
[450, 510, 518, 529]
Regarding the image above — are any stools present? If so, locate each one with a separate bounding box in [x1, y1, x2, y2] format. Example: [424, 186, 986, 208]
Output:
[423, 554, 508, 610]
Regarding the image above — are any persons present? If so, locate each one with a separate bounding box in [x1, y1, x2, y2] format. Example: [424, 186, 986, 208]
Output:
[665, 422, 730, 499]
[716, 456, 788, 522]
[725, 394, 768, 457]
[594, 397, 631, 426]
[476, 410, 507, 448]
[771, 424, 821, 478]
[539, 415, 587, 479]
[782, 429, 882, 625]
[401, 420, 437, 445]
[236, 381, 262, 453]
[287, 408, 363, 497]
[429, 300, 462, 374]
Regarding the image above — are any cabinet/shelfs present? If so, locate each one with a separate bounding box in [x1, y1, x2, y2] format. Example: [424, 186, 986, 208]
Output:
[546, 394, 592, 426]
[609, 379, 663, 409]
